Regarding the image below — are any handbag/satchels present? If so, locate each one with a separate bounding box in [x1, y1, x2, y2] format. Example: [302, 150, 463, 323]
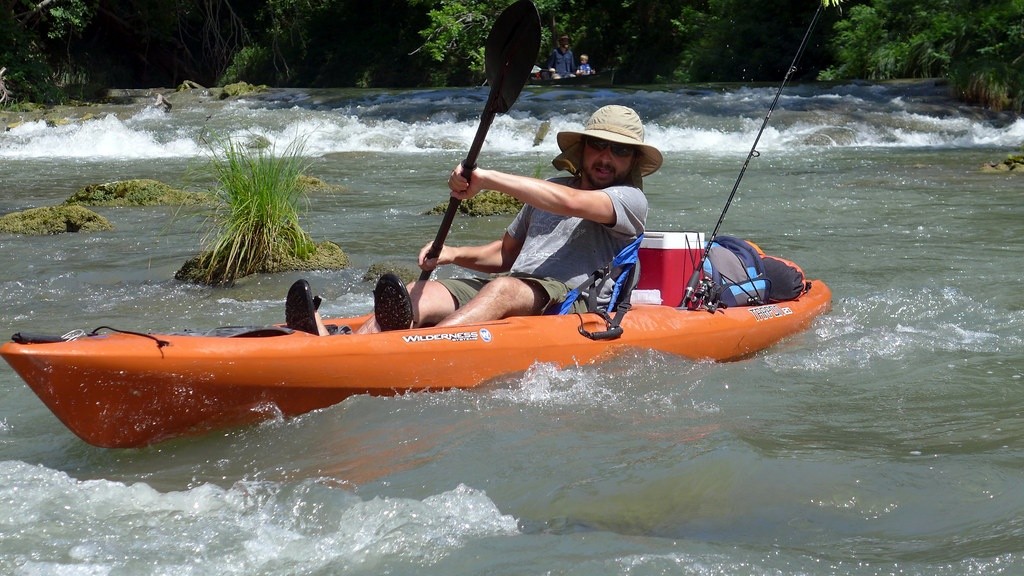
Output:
[701, 235, 771, 306]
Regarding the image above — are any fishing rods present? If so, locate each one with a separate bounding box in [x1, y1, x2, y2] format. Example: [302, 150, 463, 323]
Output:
[675, 1, 826, 310]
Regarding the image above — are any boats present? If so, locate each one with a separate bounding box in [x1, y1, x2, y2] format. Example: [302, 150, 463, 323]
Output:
[0, 241, 833, 450]
[527, 70, 616, 88]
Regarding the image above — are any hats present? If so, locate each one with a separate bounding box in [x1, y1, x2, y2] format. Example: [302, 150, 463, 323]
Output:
[552, 105, 663, 176]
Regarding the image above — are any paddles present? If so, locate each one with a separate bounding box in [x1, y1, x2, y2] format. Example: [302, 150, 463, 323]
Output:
[419, 0, 542, 283]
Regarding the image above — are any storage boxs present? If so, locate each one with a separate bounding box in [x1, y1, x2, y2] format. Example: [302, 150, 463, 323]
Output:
[634, 232, 705, 306]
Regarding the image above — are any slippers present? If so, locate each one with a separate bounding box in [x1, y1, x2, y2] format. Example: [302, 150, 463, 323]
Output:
[285, 279, 322, 336]
[372, 273, 414, 332]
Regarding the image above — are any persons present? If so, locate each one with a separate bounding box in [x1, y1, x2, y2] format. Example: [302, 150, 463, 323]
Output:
[547, 36, 591, 79]
[284, 103, 664, 336]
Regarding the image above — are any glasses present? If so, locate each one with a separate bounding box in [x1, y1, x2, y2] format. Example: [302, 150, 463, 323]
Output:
[585, 136, 638, 158]
